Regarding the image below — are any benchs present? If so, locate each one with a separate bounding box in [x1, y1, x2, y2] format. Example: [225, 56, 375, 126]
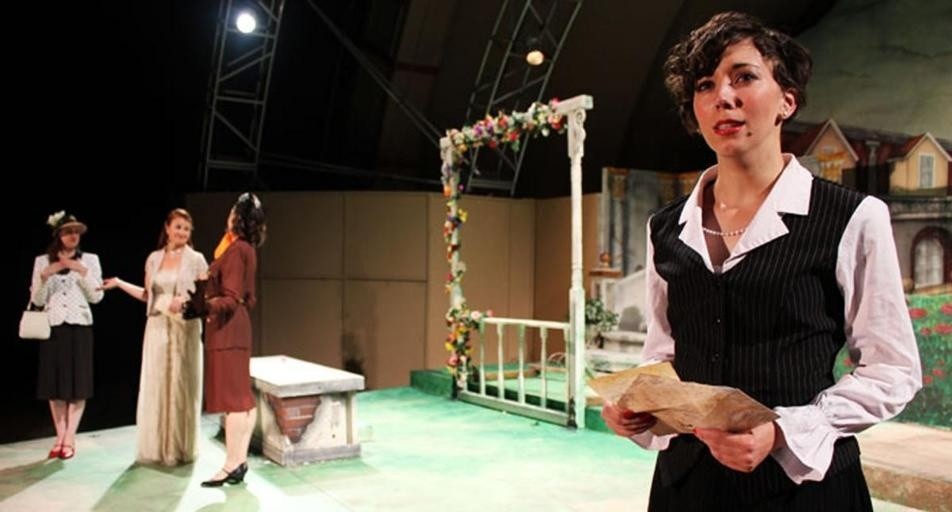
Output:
[214, 354, 365, 464]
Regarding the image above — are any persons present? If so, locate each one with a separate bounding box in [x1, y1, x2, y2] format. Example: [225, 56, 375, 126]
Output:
[94, 207, 210, 468]
[601, 11, 924, 512]
[28, 209, 105, 459]
[178, 188, 269, 489]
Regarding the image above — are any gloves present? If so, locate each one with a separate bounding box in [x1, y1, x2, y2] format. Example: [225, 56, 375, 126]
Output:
[183, 289, 207, 320]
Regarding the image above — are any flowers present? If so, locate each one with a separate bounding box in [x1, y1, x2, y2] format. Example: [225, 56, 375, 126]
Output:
[442, 96, 566, 158]
[438, 143, 494, 394]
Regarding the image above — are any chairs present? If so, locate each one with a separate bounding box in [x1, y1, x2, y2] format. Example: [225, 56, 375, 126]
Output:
[579, 267, 652, 360]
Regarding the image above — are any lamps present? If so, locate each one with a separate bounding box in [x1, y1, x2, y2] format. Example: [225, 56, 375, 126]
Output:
[525, 49, 546, 69]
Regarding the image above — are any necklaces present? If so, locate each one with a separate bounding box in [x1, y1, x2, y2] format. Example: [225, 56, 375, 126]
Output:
[700, 225, 749, 237]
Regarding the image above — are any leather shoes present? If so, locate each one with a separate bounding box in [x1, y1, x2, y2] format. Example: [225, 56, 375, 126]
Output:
[239, 461, 248, 481]
[49, 445, 62, 458]
[61, 444, 74, 459]
[201, 467, 242, 487]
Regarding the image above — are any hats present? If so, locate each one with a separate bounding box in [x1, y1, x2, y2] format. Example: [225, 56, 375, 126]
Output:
[52, 213, 88, 238]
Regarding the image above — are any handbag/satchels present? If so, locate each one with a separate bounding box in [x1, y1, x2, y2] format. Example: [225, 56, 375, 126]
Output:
[18, 300, 52, 340]
